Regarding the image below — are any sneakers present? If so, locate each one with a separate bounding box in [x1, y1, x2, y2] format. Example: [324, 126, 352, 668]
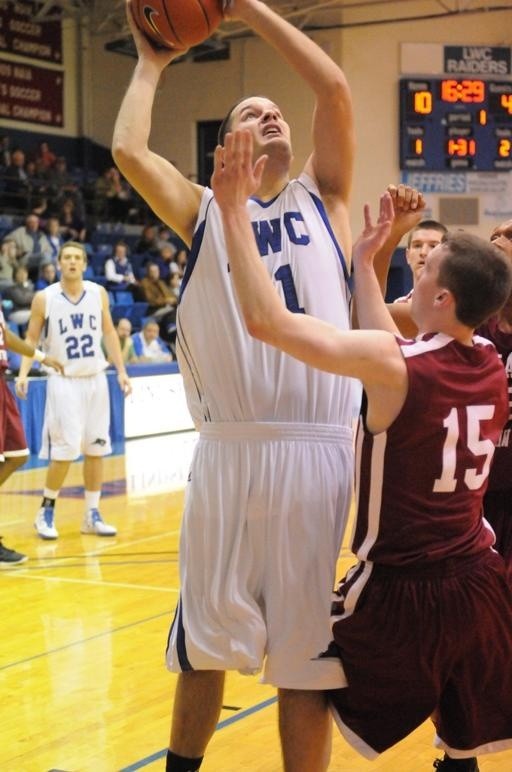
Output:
[36, 507, 58, 539]
[81, 507, 116, 535]
[1, 543, 28, 566]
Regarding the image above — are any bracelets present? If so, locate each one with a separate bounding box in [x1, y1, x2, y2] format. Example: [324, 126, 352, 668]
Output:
[32, 349, 46, 362]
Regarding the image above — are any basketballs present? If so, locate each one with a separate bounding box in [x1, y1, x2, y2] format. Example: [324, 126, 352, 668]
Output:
[130, 1, 224, 49]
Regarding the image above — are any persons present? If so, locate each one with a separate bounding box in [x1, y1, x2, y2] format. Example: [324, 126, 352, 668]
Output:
[0, 302, 65, 566]
[14, 241, 134, 540]
[353, 184, 512, 559]
[210, 127, 512, 771]
[1, 141, 189, 372]
[113, 1, 362, 770]
[374, 184, 511, 303]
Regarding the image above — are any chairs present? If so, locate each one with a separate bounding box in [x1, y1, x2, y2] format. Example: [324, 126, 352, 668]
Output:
[0, 128, 199, 377]
